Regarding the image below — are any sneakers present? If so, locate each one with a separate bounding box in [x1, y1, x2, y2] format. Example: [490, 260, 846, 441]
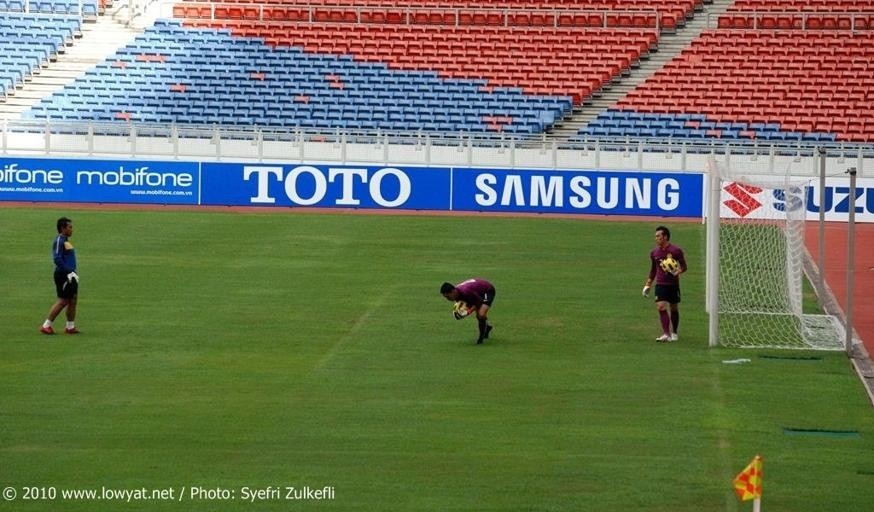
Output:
[656, 333, 669, 341]
[477, 326, 492, 344]
[670, 333, 677, 341]
[66, 326, 79, 333]
[40, 324, 55, 335]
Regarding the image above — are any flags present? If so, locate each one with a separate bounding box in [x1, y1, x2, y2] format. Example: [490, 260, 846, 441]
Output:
[733, 459, 764, 501]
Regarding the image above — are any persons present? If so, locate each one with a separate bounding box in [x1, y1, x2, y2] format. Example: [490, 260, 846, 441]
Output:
[641, 226, 688, 343]
[440, 278, 496, 344]
[40, 216, 81, 335]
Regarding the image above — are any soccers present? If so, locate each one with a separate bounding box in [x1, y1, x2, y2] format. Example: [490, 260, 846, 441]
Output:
[660, 258, 677, 273]
[453, 301, 466, 313]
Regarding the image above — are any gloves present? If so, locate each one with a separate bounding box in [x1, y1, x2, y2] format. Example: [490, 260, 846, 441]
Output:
[642, 285, 651, 299]
[68, 272, 79, 285]
[454, 310, 468, 321]
